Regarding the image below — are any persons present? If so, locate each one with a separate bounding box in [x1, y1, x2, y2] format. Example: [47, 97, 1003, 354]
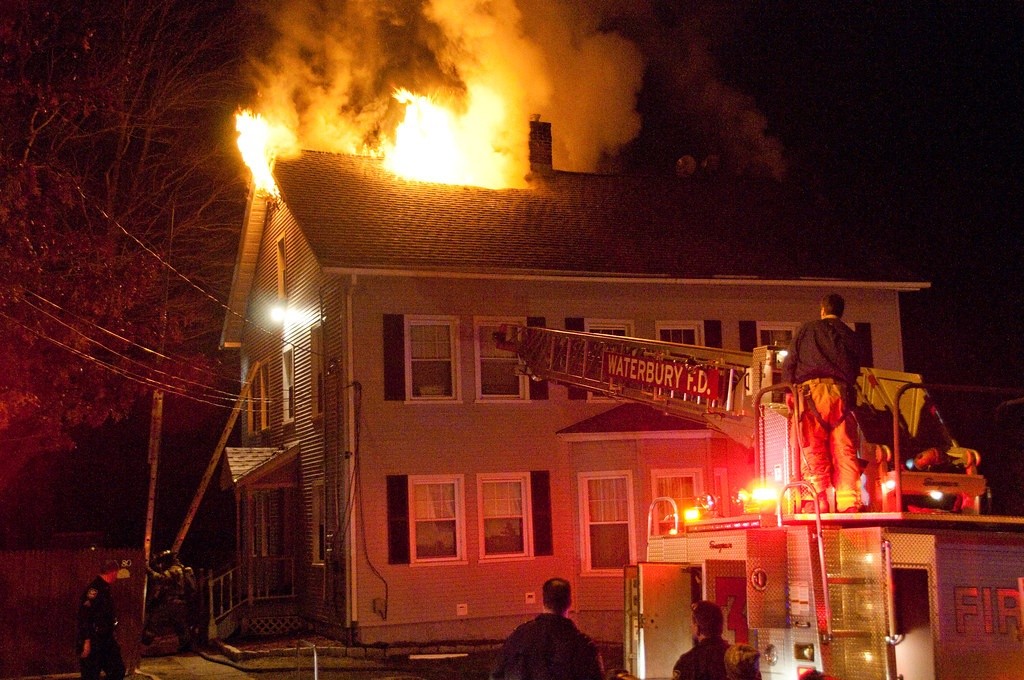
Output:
[141, 550, 194, 654]
[779, 293, 861, 513]
[76, 559, 126, 680]
[723, 643, 760, 680]
[672, 600, 730, 680]
[489, 577, 604, 680]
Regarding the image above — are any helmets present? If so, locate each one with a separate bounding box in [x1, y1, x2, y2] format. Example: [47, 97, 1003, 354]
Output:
[157, 548, 178, 562]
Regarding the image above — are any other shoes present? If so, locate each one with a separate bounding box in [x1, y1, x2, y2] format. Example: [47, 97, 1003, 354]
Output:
[804, 499, 829, 513]
[178, 645, 193, 657]
[141, 646, 153, 657]
[841, 504, 864, 513]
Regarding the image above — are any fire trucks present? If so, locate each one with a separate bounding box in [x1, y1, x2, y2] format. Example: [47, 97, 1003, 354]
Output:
[489, 319, 1024, 679]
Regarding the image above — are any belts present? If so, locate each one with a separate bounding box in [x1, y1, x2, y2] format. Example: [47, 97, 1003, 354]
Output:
[801, 378, 848, 385]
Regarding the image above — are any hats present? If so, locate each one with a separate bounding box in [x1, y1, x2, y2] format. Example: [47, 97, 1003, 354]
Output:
[724, 644, 761, 680]
